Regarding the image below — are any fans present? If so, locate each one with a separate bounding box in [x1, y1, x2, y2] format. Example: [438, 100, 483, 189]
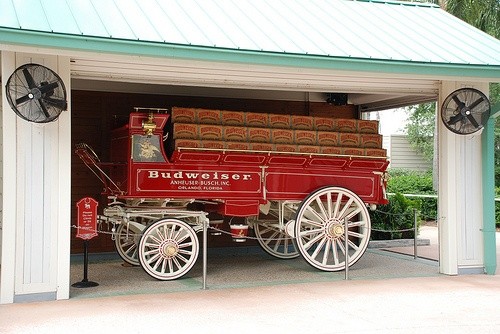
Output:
[4, 63, 68, 126]
[441, 87, 491, 135]
